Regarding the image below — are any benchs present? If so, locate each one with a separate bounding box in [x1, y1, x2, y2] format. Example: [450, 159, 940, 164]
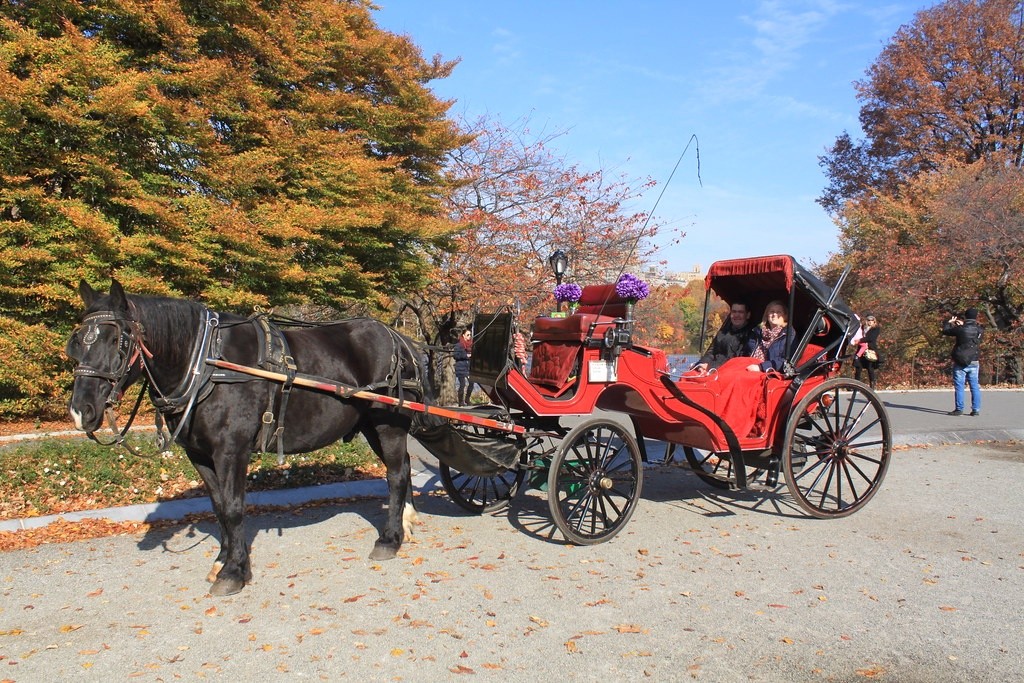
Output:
[531, 283, 629, 342]
[680, 344, 828, 418]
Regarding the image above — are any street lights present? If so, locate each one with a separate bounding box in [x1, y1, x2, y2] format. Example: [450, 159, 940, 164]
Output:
[549, 249, 568, 312]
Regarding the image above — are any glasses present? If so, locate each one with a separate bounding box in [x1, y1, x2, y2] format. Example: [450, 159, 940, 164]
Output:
[467, 334, 472, 336]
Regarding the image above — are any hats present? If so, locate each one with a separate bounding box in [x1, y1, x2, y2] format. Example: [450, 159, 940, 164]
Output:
[965, 308, 978, 319]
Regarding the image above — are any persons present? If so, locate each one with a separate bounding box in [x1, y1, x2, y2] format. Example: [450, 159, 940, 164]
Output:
[453, 329, 474, 407]
[694, 299, 796, 374]
[942, 305, 984, 417]
[849, 313, 884, 392]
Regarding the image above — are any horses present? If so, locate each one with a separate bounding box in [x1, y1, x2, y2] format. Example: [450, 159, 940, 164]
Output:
[64, 278, 446, 595]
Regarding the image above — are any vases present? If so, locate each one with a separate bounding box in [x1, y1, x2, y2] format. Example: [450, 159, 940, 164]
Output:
[568, 306, 575, 316]
[624, 301, 634, 329]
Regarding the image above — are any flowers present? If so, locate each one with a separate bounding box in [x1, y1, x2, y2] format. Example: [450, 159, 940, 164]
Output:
[615, 273, 649, 305]
[552, 282, 583, 305]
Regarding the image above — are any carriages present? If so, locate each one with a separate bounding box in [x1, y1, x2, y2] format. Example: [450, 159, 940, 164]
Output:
[66, 254, 892, 597]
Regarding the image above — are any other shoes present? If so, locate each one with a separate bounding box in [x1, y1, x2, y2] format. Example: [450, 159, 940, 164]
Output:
[459, 402, 466, 406]
[465, 399, 473, 406]
[970, 412, 979, 416]
[948, 410, 963, 416]
[854, 355, 858, 361]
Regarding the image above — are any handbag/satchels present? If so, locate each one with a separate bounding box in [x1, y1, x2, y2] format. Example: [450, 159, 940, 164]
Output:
[864, 349, 878, 362]
